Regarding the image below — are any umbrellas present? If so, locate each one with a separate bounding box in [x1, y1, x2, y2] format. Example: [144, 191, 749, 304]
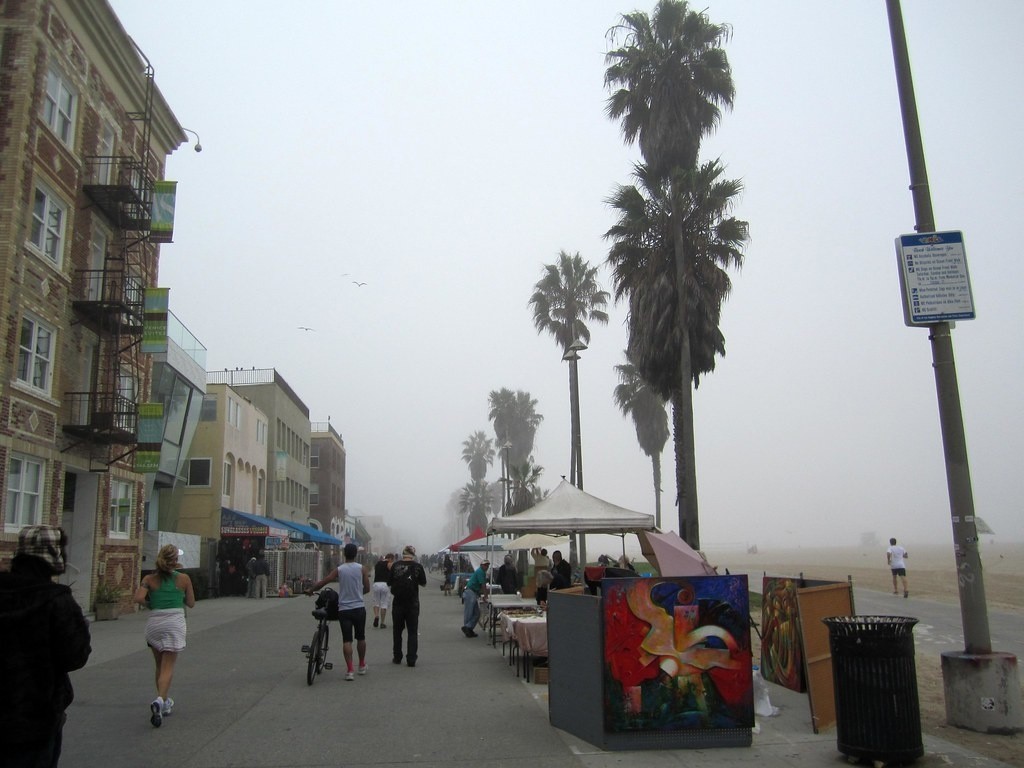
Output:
[643, 530, 718, 576]
[501, 533, 573, 552]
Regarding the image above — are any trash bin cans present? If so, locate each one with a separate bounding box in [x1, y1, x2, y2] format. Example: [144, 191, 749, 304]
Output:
[822, 615, 924, 760]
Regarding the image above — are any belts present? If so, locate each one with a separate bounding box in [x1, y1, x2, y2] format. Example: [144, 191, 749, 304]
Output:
[470, 588, 476, 592]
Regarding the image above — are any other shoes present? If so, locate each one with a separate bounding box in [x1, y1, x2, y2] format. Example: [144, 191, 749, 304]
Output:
[468, 628, 478, 637]
[393, 658, 401, 664]
[894, 591, 898, 594]
[407, 661, 415, 667]
[451, 586, 454, 589]
[380, 624, 386, 628]
[461, 626, 475, 636]
[374, 617, 379, 627]
[904, 591, 909, 598]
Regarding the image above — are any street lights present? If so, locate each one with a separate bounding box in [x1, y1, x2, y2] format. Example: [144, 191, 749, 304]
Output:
[561, 321, 590, 588]
[502, 440, 515, 555]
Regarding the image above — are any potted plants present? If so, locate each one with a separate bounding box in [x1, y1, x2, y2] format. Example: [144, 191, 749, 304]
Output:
[92, 577, 125, 620]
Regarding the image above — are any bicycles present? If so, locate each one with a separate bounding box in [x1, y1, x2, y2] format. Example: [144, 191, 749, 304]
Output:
[300, 587, 334, 687]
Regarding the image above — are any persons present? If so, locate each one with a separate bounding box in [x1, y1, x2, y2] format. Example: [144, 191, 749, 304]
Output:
[372, 554, 442, 573]
[495, 555, 519, 594]
[245, 552, 270, 600]
[373, 554, 394, 629]
[614, 555, 635, 572]
[887, 537, 909, 598]
[444, 554, 467, 589]
[134, 544, 195, 727]
[531, 547, 552, 576]
[549, 551, 570, 590]
[305, 543, 370, 680]
[387, 545, 427, 667]
[0, 525, 92, 768]
[461, 560, 490, 638]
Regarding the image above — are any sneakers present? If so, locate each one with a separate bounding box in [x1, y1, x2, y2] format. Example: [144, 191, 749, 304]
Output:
[150, 700, 162, 728]
[345, 672, 354, 680]
[162, 698, 175, 715]
[358, 664, 368, 674]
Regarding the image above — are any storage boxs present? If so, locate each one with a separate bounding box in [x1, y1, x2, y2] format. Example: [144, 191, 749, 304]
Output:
[530, 656, 548, 684]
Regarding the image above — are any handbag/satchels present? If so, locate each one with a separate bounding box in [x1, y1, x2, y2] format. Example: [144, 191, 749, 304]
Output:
[315, 587, 339, 621]
[444, 571, 448, 575]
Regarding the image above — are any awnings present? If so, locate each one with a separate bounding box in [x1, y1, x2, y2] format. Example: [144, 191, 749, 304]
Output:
[220, 506, 304, 540]
[274, 517, 342, 545]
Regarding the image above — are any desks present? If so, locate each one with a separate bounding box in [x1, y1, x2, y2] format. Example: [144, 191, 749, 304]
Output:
[451, 572, 548, 683]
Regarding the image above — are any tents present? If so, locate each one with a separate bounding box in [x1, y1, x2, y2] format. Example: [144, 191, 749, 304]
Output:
[457, 534, 513, 578]
[486, 479, 655, 639]
[438, 543, 469, 571]
[449, 527, 487, 573]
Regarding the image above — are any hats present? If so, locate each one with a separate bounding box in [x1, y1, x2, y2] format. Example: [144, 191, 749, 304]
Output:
[17, 526, 66, 575]
[481, 559, 490, 564]
[251, 557, 256, 561]
